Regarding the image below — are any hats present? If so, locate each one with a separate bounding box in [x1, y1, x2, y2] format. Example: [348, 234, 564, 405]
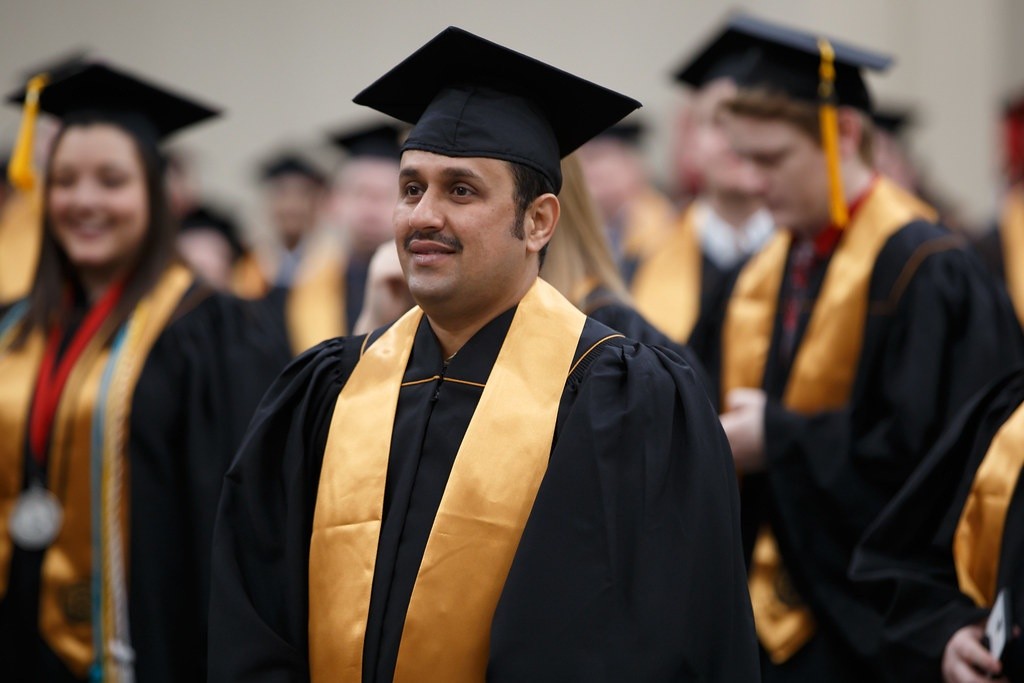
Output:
[7, 61, 222, 192]
[351, 26, 642, 197]
[672, 19, 893, 224]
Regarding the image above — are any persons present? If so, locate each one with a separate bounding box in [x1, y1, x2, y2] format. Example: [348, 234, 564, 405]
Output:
[870, 109, 962, 237]
[683, 13, 1024, 678]
[210, 23, 759, 683]
[1, 64, 282, 682]
[844, 358, 1024, 682]
[149, 28, 777, 358]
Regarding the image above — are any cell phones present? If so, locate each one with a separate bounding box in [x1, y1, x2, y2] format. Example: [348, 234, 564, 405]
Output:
[973, 586, 1011, 676]
